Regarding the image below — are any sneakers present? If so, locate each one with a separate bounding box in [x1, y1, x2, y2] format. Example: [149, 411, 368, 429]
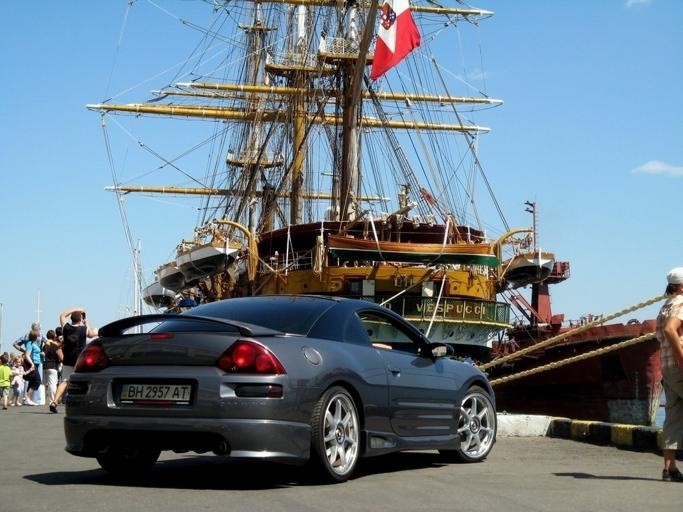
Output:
[661, 466, 683, 483]
[0, 396, 39, 410]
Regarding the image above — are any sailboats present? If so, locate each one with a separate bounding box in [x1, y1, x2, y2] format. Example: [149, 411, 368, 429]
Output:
[83, 1, 514, 367]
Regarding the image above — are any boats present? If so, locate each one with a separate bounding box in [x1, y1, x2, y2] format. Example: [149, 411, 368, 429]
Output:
[474, 201, 665, 427]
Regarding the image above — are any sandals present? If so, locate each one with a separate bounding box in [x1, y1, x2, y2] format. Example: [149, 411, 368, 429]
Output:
[49, 402, 58, 414]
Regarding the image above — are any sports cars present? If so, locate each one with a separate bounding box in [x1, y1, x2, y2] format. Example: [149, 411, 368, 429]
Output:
[61, 295, 499, 480]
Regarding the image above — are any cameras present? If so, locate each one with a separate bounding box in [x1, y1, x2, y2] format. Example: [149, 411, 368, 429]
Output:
[81, 312, 86, 319]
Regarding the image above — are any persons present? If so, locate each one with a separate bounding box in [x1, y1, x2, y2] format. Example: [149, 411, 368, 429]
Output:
[649, 266, 683, 484]
[0, 306, 98, 414]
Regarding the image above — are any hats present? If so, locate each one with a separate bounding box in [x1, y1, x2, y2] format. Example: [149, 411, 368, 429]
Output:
[667, 267, 683, 284]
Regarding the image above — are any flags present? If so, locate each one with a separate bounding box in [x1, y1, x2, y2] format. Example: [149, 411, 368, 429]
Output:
[368, 0, 423, 82]
